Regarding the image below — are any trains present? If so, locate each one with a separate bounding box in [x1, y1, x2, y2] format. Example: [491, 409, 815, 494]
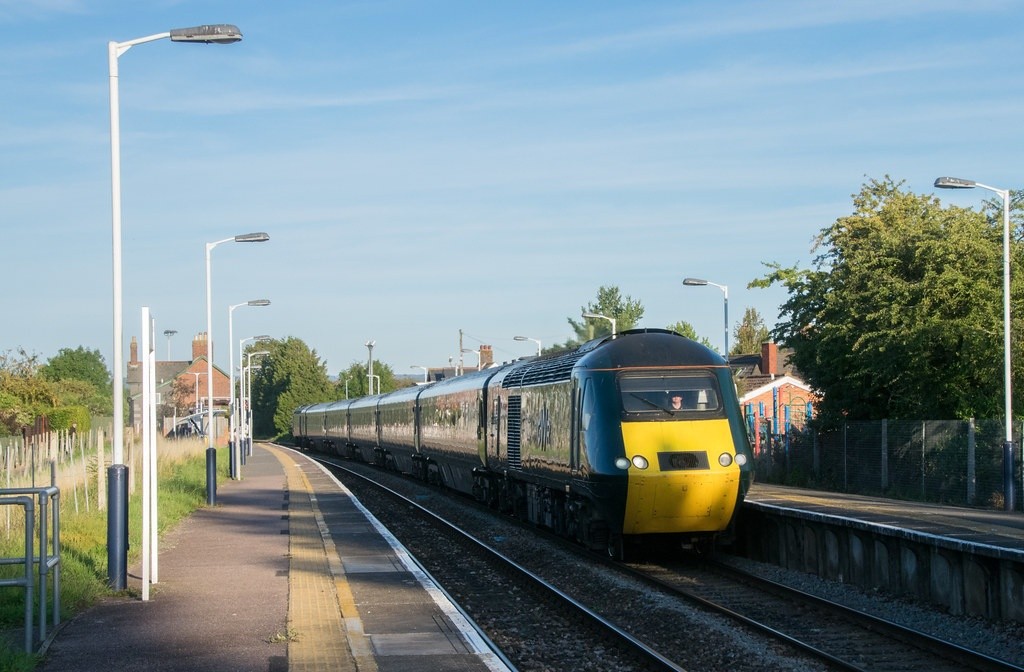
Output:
[293, 329, 755, 562]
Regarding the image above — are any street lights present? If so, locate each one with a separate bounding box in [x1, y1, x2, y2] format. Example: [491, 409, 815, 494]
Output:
[682, 278, 728, 361]
[244, 366, 262, 449]
[461, 349, 480, 370]
[410, 366, 428, 382]
[366, 375, 380, 395]
[345, 376, 352, 399]
[513, 336, 541, 357]
[205, 232, 269, 507]
[582, 313, 616, 339]
[241, 335, 268, 466]
[228, 300, 270, 479]
[934, 177, 1017, 512]
[105, 23, 242, 589]
[248, 351, 268, 456]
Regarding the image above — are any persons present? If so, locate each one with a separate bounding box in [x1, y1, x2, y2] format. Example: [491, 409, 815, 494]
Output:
[668, 391, 690, 409]
[68, 422, 78, 449]
